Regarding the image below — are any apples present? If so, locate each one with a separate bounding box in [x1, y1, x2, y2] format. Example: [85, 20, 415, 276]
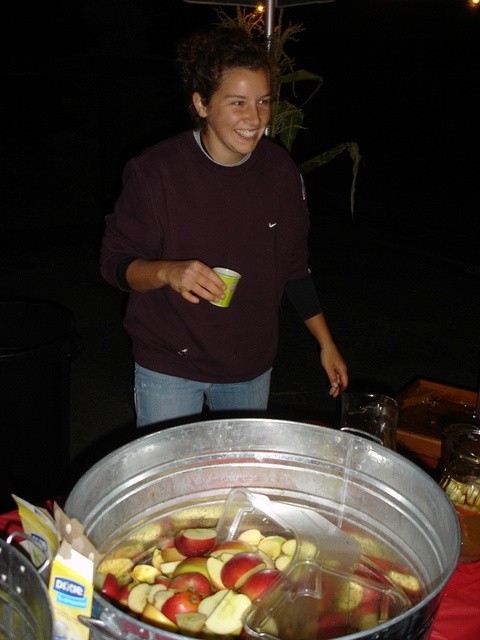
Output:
[100, 526, 424, 638]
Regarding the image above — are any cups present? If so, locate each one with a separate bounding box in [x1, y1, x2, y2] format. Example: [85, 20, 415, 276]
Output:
[341, 391, 400, 453]
[208, 267, 241, 308]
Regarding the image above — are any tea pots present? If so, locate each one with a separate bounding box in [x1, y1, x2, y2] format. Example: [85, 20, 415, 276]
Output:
[439, 422, 480, 563]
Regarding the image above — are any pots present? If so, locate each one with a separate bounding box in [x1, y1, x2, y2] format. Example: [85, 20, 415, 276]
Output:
[1, 531, 54, 640]
[63, 417, 463, 639]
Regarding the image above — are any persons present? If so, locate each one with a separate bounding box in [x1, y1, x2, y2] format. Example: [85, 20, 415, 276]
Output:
[98, 51, 350, 428]
